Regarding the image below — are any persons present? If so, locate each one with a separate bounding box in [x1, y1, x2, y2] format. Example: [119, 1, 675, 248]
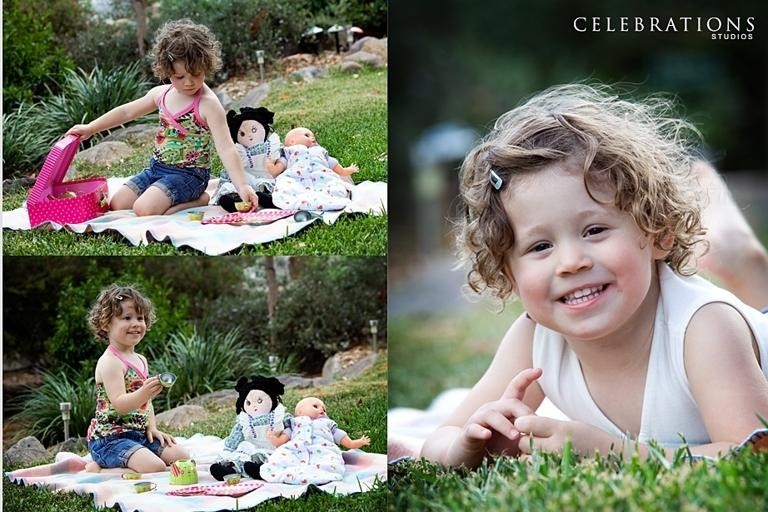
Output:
[217, 107, 282, 212]
[265, 127, 359, 211]
[85, 284, 188, 473]
[388, 78, 768, 471]
[65, 18, 260, 216]
[209, 375, 294, 481]
[260, 397, 371, 486]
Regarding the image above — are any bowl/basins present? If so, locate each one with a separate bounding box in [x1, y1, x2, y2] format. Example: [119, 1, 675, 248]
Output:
[223, 473, 241, 487]
[187, 211, 204, 220]
[234, 201, 253, 212]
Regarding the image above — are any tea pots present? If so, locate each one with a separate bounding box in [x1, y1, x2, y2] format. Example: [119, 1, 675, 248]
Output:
[170, 458, 198, 485]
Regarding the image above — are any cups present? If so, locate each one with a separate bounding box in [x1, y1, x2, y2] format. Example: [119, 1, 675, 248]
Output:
[134, 482, 157, 494]
[121, 473, 141, 480]
[158, 372, 178, 388]
